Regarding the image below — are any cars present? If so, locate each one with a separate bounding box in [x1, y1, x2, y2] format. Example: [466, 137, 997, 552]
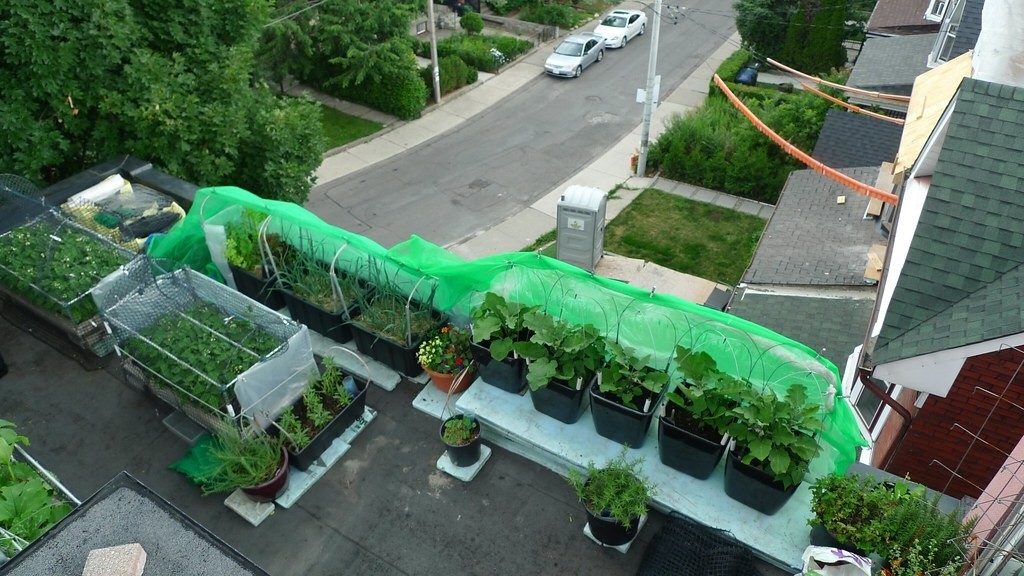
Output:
[593, 9, 648, 49]
[543, 32, 607, 79]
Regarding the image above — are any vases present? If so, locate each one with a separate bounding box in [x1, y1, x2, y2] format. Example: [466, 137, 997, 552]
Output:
[421, 365, 477, 393]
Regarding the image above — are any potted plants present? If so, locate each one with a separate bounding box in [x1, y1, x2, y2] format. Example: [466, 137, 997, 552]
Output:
[588, 336, 671, 450]
[723, 367, 828, 516]
[465, 292, 546, 394]
[778, 82, 793, 93]
[439, 414, 482, 467]
[216, 208, 311, 311]
[512, 310, 609, 425]
[657, 344, 754, 480]
[260, 225, 380, 344]
[192, 416, 290, 503]
[805, 473, 980, 576]
[264, 355, 372, 472]
[327, 255, 449, 379]
[0, 209, 159, 355]
[0, 418, 82, 571]
[121, 298, 318, 443]
[565, 442, 658, 546]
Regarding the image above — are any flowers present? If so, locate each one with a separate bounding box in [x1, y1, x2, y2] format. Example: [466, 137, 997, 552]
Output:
[414, 322, 478, 377]
